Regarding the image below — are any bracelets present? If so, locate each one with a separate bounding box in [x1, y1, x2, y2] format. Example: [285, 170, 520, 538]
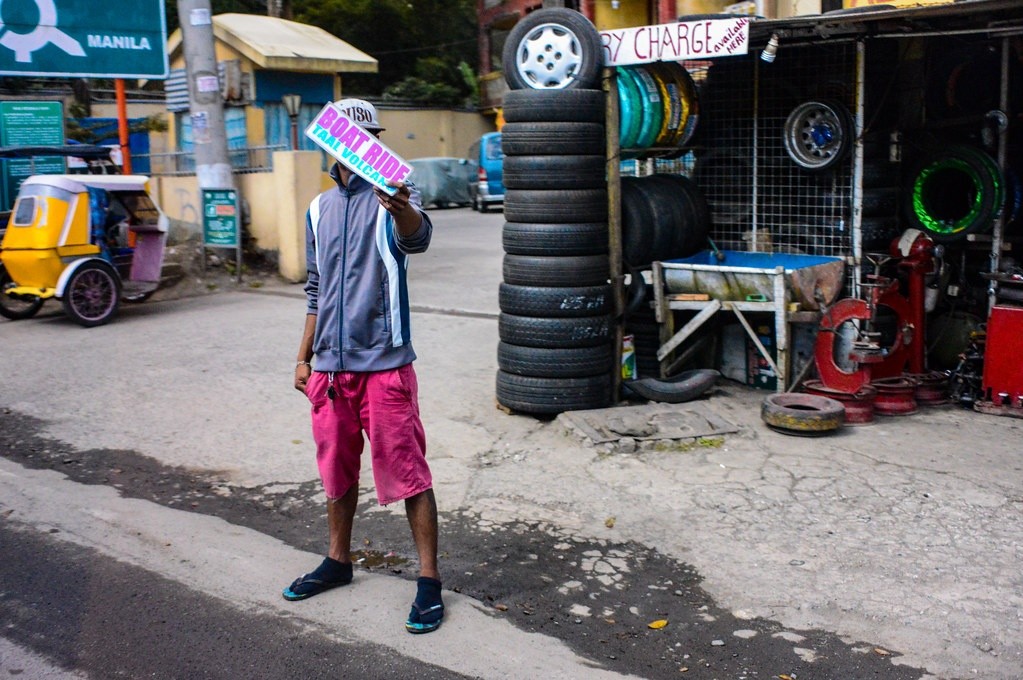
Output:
[295, 361, 308, 366]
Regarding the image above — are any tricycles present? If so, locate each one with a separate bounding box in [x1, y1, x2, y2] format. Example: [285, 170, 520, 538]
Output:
[0, 146, 133, 211]
[0, 174, 168, 329]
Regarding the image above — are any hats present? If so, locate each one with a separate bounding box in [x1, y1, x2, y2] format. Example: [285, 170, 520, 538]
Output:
[334, 98, 386, 134]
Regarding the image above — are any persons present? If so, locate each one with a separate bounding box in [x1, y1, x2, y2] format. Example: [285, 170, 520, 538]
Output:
[282, 97, 444, 634]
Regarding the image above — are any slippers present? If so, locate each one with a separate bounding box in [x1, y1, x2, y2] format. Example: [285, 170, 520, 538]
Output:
[281, 572, 351, 600]
[406, 602, 444, 633]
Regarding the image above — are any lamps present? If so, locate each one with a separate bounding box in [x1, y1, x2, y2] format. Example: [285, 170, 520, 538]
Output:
[762, 34, 780, 62]
[282, 89, 300, 123]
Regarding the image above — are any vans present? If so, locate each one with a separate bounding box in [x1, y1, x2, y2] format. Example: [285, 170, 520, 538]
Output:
[459, 131, 506, 215]
